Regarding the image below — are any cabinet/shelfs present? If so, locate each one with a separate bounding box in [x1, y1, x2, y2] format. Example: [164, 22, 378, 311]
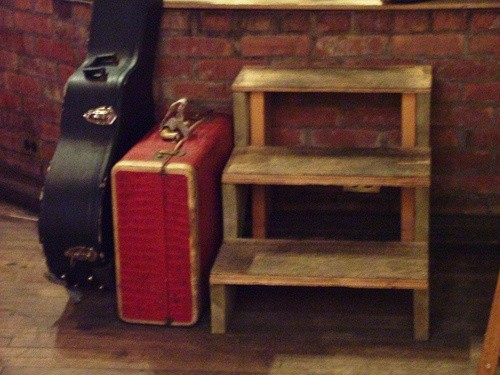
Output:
[206, 63, 434, 343]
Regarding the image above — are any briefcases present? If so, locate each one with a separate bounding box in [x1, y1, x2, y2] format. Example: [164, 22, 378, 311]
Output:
[110, 96, 234, 327]
